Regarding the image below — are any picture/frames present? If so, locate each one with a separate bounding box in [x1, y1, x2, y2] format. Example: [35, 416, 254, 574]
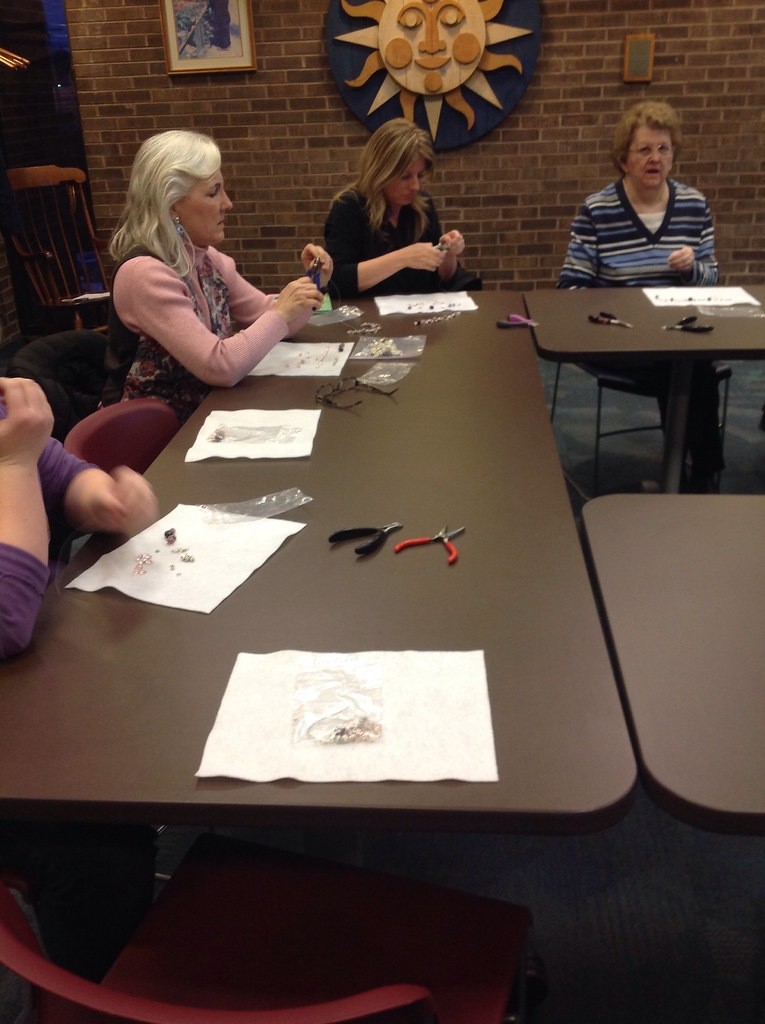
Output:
[159, 0, 258, 75]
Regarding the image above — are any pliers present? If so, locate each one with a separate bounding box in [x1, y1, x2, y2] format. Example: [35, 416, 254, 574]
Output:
[432, 241, 451, 254]
[662, 316, 714, 333]
[305, 257, 321, 311]
[497, 314, 540, 329]
[328, 522, 404, 555]
[394, 524, 466, 565]
[588, 312, 634, 328]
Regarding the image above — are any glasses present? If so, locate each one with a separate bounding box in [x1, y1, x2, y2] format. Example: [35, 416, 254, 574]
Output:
[626, 141, 677, 156]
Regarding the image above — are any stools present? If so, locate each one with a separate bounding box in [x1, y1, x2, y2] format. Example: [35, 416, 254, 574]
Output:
[593, 364, 732, 490]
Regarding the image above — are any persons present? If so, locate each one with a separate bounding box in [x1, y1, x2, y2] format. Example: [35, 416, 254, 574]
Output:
[322, 114, 483, 297]
[556, 98, 727, 494]
[1, 375, 159, 663]
[99, 128, 334, 433]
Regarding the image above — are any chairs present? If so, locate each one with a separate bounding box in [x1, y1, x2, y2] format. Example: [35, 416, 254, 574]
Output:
[58, 397, 180, 476]
[0, 832, 533, 1024]
[0, 165, 116, 333]
[10, 333, 128, 441]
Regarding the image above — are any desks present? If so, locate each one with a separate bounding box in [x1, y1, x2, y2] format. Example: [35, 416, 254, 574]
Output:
[0, 286, 641, 830]
[579, 492, 765, 836]
[522, 285, 765, 491]
